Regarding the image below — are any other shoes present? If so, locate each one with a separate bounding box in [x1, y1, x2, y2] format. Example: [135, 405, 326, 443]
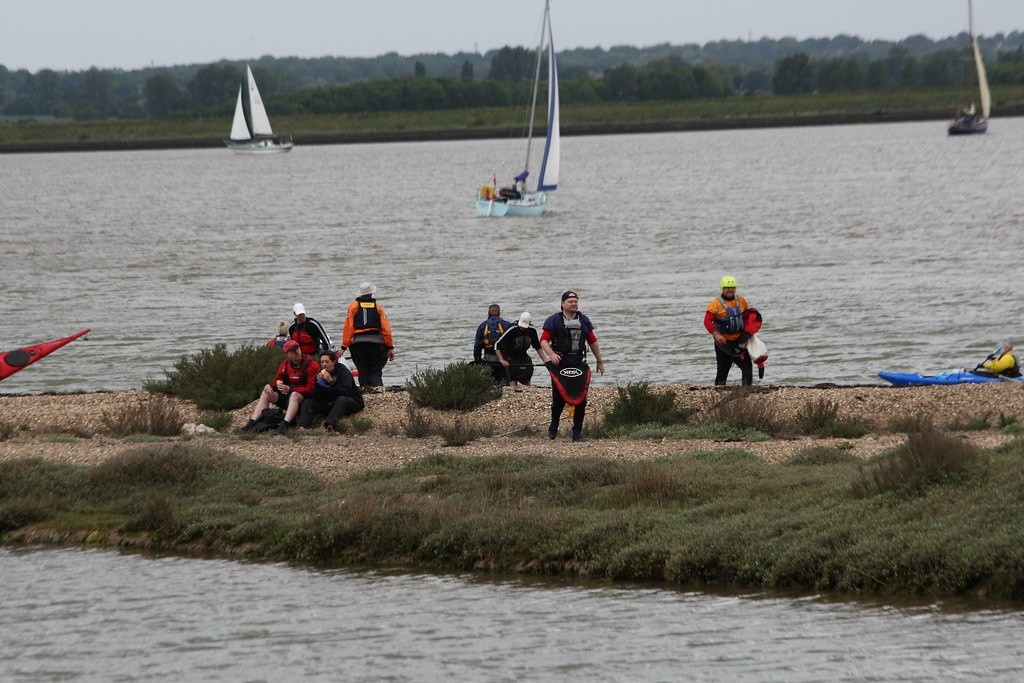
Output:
[242, 420, 254, 431]
[274, 423, 286, 435]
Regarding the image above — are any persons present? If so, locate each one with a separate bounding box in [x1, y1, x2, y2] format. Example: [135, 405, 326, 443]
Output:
[334, 282, 394, 391]
[494, 312, 547, 386]
[703, 276, 753, 386]
[539, 291, 605, 441]
[288, 302, 330, 356]
[266, 320, 288, 347]
[474, 304, 511, 386]
[240, 340, 321, 435]
[296, 350, 365, 433]
[961, 338, 1019, 378]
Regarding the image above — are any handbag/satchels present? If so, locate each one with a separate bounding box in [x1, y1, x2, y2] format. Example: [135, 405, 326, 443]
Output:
[338, 355, 351, 372]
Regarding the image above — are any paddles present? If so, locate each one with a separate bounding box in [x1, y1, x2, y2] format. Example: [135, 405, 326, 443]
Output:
[468, 360, 545, 368]
[972, 342, 1005, 372]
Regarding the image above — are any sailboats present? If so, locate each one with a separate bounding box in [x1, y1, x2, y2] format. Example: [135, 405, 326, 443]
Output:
[947, 1, 991, 136]
[223, 65, 293, 155]
[476, 0, 562, 218]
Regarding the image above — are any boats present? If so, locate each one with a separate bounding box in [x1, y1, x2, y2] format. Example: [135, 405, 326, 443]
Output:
[878, 369, 1024, 387]
[0, 327, 93, 381]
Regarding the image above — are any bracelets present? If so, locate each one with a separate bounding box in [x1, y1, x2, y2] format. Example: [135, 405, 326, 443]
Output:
[597, 362, 603, 364]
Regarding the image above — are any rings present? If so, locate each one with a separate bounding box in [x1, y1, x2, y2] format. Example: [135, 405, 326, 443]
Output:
[323, 375, 325, 376]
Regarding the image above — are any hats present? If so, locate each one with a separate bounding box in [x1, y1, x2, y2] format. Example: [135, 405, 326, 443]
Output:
[293, 303, 306, 316]
[355, 283, 377, 295]
[283, 340, 299, 353]
[560, 291, 578, 310]
[518, 312, 531, 328]
[278, 321, 289, 335]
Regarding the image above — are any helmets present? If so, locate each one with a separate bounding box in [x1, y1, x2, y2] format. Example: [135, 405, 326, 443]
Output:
[720, 276, 737, 290]
